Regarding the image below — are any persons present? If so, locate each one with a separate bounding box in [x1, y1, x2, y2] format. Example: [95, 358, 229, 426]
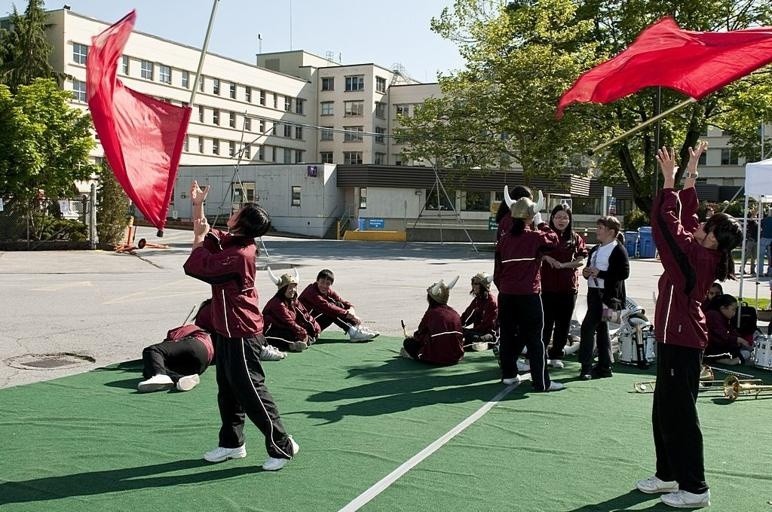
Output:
[637, 141, 745, 509]
[299, 269, 380, 343]
[183, 180, 300, 471]
[460, 272, 500, 352]
[191, 297, 287, 361]
[493, 185, 567, 392]
[541, 203, 589, 368]
[262, 267, 322, 352]
[496, 185, 538, 372]
[699, 206, 772, 366]
[403, 276, 465, 367]
[578, 215, 629, 380]
[137, 324, 214, 393]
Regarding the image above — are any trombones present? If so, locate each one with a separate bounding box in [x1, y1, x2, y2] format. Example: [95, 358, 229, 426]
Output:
[634, 366, 772, 400]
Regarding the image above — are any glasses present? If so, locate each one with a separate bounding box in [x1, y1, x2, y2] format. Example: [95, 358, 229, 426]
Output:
[553, 216, 570, 221]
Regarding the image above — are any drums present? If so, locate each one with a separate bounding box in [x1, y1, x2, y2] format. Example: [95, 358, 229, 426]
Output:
[753, 335, 772, 371]
[618, 335, 656, 365]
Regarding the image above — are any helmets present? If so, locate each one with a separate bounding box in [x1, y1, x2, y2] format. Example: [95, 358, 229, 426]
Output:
[426, 282, 449, 303]
[511, 197, 539, 218]
[471, 273, 492, 291]
[278, 273, 298, 289]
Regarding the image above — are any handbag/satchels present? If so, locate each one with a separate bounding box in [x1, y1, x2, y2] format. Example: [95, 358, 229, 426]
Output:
[602, 302, 622, 324]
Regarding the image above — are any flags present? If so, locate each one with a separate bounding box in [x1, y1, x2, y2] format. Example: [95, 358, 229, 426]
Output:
[86, 10, 192, 230]
[559, 17, 772, 111]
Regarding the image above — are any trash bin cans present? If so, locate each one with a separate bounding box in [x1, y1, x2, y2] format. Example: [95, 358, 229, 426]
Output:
[638, 227, 656, 258]
[624, 231, 639, 257]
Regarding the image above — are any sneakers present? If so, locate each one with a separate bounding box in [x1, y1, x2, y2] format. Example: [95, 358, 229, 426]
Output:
[503, 358, 530, 384]
[547, 359, 564, 368]
[290, 340, 307, 352]
[400, 348, 414, 360]
[471, 340, 488, 350]
[263, 437, 300, 470]
[261, 345, 287, 360]
[350, 326, 381, 342]
[204, 442, 247, 462]
[581, 366, 612, 380]
[637, 475, 711, 507]
[542, 381, 563, 390]
[138, 373, 200, 392]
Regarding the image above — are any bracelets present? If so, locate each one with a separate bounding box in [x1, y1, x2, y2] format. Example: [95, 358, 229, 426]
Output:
[684, 171, 699, 179]
